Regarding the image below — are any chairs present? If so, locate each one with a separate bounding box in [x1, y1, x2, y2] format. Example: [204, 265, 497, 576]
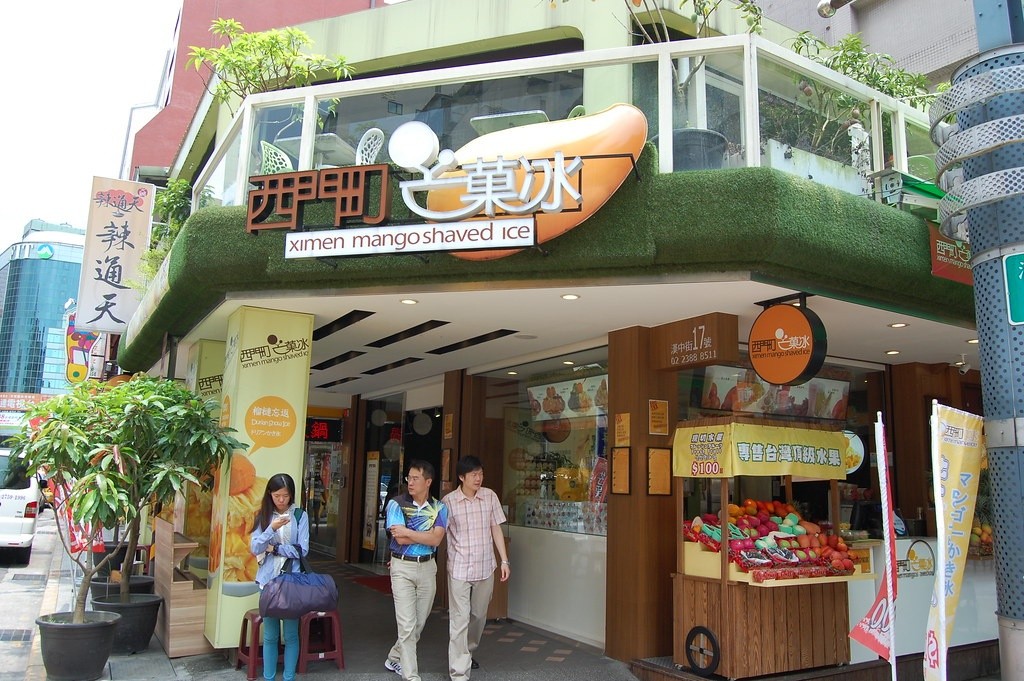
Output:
[260, 140, 295, 175]
[650, 128, 728, 172]
[568, 105, 584, 120]
[322, 128, 385, 171]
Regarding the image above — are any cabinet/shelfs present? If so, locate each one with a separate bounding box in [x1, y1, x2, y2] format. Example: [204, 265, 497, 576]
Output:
[667, 539, 856, 680]
[153, 515, 225, 658]
[444, 537, 510, 622]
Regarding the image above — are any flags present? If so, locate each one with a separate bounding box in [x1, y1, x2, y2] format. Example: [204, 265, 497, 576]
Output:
[923, 405, 984, 681]
[848, 423, 897, 667]
[587, 457, 608, 516]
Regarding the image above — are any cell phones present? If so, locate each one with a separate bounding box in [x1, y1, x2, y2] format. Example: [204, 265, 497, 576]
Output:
[279, 514, 290, 522]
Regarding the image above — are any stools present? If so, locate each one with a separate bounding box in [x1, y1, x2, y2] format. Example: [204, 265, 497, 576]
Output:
[235, 608, 285, 681]
[297, 610, 343, 674]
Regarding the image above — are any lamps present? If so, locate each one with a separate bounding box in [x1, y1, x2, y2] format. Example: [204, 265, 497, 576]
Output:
[435, 408, 441, 417]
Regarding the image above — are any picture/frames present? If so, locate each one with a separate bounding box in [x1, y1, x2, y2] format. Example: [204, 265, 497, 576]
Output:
[646, 446, 673, 496]
[611, 446, 632, 495]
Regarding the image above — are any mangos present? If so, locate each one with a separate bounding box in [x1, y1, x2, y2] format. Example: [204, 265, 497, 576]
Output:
[969, 523, 993, 547]
[727, 498, 857, 571]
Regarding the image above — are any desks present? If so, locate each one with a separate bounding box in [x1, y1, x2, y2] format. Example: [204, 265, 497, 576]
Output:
[470, 111, 550, 138]
[274, 132, 356, 170]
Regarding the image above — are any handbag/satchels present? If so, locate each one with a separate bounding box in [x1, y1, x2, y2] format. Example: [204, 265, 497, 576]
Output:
[259, 544, 338, 620]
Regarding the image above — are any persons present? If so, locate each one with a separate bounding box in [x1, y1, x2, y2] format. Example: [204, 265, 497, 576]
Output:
[249, 473, 338, 681]
[440, 455, 510, 681]
[306, 471, 325, 536]
[385, 460, 448, 681]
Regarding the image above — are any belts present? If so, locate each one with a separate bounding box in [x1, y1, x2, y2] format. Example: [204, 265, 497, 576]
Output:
[392, 552, 436, 562]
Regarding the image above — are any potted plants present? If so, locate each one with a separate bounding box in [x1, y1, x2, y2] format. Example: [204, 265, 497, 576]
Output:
[538, 0, 769, 170]
[722, 30, 956, 198]
[0, 371, 249, 680]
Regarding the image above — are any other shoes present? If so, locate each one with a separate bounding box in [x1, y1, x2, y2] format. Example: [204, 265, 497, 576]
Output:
[385, 659, 402, 675]
[471, 659, 479, 668]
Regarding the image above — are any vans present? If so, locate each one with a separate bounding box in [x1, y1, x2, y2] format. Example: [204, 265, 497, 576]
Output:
[1, 447, 49, 565]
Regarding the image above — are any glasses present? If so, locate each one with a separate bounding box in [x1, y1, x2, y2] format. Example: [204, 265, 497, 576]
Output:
[405, 477, 419, 481]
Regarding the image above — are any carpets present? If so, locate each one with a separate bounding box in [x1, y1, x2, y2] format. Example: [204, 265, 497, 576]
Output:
[351, 574, 393, 597]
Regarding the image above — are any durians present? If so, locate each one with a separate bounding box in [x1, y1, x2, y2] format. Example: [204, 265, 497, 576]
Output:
[185, 454, 278, 585]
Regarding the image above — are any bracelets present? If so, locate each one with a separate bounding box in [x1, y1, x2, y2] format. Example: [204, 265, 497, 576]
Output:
[272, 543, 280, 556]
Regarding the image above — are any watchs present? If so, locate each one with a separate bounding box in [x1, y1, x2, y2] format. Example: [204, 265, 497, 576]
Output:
[501, 561, 510, 566]
